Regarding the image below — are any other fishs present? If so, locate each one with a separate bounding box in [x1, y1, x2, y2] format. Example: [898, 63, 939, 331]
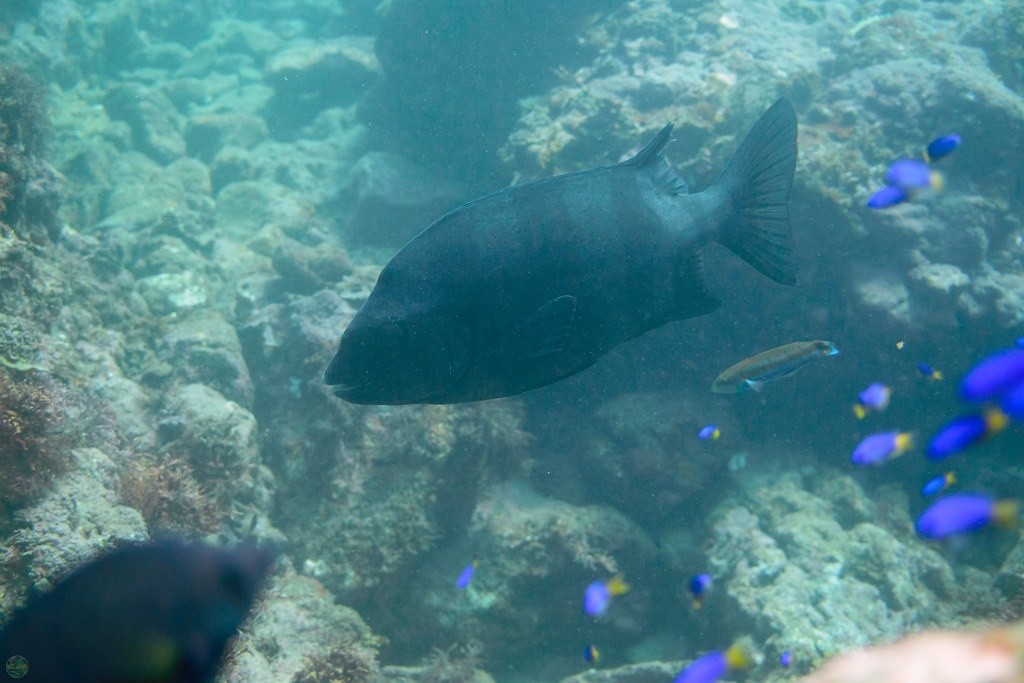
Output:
[0, 540, 276, 683]
[324, 98, 1024, 683]
